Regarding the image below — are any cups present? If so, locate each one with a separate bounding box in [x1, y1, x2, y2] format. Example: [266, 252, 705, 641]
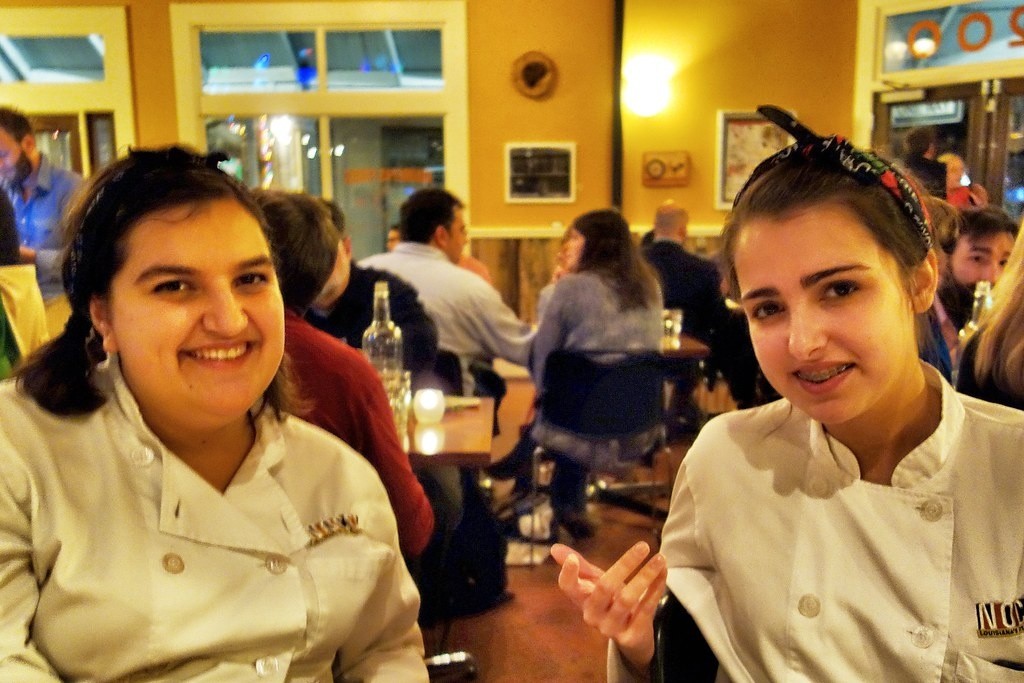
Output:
[663, 308, 682, 350]
[383, 369, 410, 424]
[413, 387, 445, 424]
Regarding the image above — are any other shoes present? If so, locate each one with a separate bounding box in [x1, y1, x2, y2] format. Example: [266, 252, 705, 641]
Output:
[485, 448, 534, 480]
[551, 508, 600, 541]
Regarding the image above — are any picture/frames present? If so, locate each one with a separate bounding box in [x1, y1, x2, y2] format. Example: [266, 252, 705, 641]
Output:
[714, 108, 798, 212]
[503, 141, 577, 205]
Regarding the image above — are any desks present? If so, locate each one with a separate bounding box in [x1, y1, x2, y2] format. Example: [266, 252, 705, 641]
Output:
[489, 332, 708, 499]
[399, 392, 496, 466]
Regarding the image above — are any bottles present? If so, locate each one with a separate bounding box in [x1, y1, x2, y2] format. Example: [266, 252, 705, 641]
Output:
[953, 282, 993, 390]
[362, 282, 402, 374]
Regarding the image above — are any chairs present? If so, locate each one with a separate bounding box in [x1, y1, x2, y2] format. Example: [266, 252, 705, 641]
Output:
[422, 347, 720, 683]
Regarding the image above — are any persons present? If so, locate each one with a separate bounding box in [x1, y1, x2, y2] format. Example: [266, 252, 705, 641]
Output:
[0, 144, 428, 683]
[904, 125, 1024, 409]
[252, 187, 440, 559]
[550, 105, 1024, 683]
[353, 190, 665, 538]
[0, 107, 84, 300]
[643, 201, 757, 419]
